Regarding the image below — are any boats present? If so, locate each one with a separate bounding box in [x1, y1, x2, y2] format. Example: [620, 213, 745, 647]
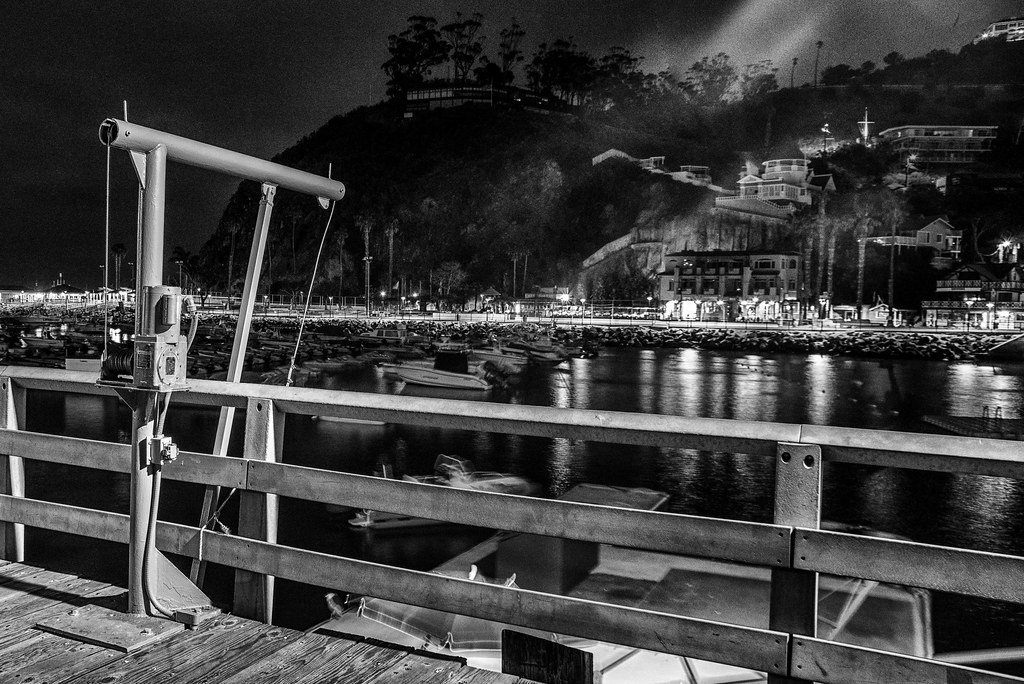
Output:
[347, 453, 533, 534]
[379, 339, 564, 391]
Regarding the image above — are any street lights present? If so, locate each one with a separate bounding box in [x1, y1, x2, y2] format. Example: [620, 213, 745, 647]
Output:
[362, 256, 373, 318]
